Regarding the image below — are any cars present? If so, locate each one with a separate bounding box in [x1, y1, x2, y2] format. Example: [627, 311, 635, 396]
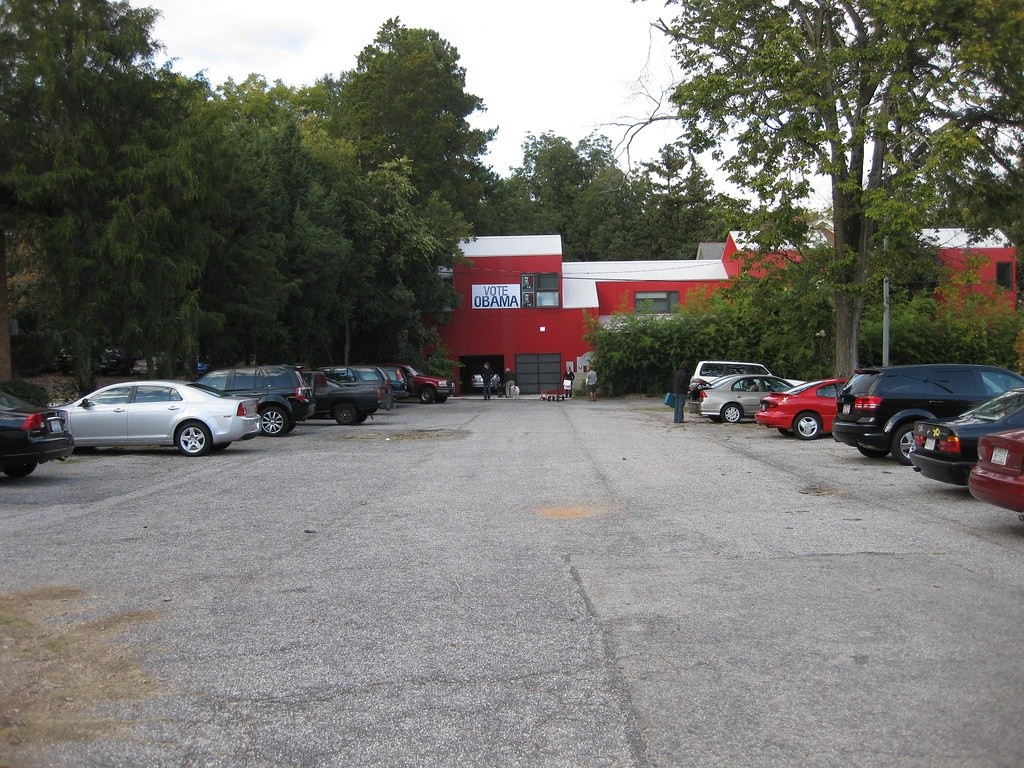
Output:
[1, 392, 74, 478]
[907, 385, 1024, 489]
[687, 372, 800, 424]
[968, 427, 1024, 518]
[755, 377, 851, 441]
[298, 368, 398, 425]
[46, 381, 265, 457]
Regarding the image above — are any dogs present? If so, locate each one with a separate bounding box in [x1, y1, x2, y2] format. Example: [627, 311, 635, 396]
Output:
[510, 385, 520, 400]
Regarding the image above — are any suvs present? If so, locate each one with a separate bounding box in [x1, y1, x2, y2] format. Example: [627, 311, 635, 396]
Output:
[832, 360, 1024, 465]
[317, 365, 457, 405]
[186, 364, 319, 437]
[686, 360, 808, 426]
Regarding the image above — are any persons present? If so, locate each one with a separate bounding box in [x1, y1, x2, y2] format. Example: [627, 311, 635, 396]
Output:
[585, 366, 597, 401]
[671, 362, 693, 424]
[562, 366, 575, 398]
[497, 367, 516, 398]
[480, 361, 494, 401]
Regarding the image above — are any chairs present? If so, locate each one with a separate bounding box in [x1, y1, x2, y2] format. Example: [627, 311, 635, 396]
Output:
[757, 382, 766, 392]
